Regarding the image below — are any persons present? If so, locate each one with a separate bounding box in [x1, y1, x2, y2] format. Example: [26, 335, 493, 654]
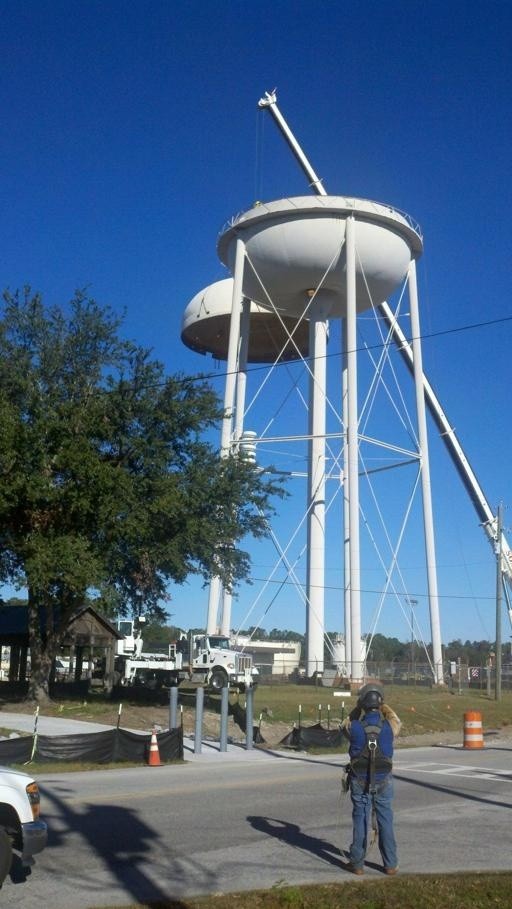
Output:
[338, 682, 403, 874]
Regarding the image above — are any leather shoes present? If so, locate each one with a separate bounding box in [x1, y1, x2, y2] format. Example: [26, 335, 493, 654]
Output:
[354, 870, 364, 875]
[385, 866, 399, 874]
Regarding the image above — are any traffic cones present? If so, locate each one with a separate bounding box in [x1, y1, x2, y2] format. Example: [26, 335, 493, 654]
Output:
[144, 729, 165, 766]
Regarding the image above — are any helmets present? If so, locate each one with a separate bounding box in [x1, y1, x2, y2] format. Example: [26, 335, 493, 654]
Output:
[358, 683, 383, 709]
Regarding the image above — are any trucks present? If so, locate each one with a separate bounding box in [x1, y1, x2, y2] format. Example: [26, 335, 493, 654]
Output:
[118, 632, 259, 693]
